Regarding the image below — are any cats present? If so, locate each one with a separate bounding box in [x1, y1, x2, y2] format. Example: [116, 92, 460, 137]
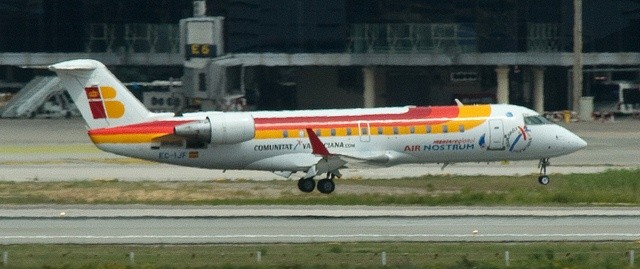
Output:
[48, 58, 588, 193]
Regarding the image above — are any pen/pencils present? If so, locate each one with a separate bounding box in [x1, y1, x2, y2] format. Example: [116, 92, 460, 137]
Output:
[593, 78, 639, 117]
